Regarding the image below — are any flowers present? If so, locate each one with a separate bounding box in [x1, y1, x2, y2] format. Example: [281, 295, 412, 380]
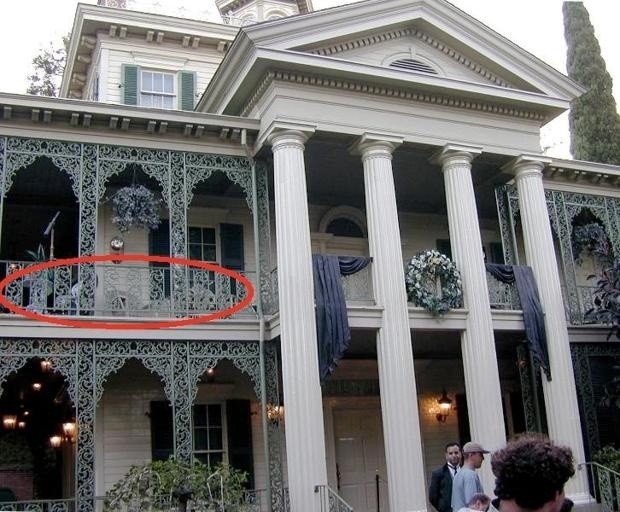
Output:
[405, 248, 462, 318]
[110, 184, 164, 235]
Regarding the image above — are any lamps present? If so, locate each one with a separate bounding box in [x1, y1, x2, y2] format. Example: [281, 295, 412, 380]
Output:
[3, 360, 75, 452]
[435, 389, 452, 423]
[267, 400, 284, 427]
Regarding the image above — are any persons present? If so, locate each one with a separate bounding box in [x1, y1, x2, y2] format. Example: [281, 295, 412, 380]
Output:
[457, 493, 490, 512]
[485, 431, 576, 512]
[428, 440, 463, 512]
[451, 441, 490, 512]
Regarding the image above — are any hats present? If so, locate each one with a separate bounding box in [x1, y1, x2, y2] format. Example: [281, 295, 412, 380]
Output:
[462, 440, 491, 455]
